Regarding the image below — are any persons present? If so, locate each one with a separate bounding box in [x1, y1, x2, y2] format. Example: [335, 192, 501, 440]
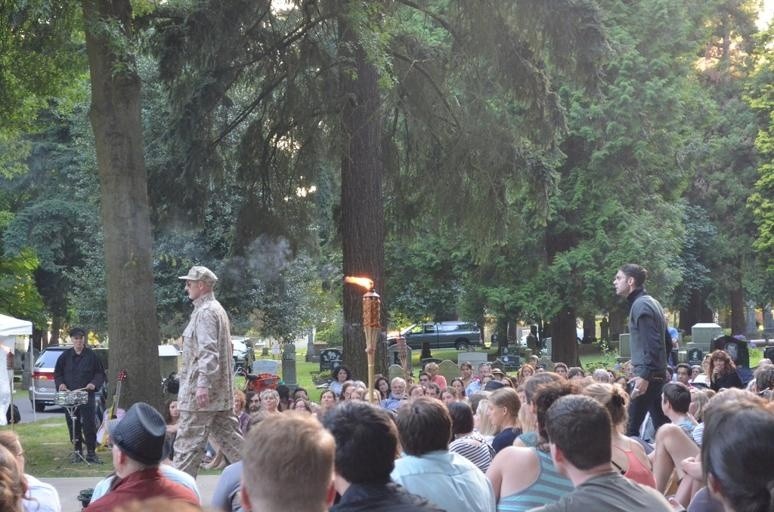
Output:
[480, 375, 493, 390]
[294, 397, 312, 414]
[89, 463, 203, 504]
[529, 354, 543, 373]
[492, 367, 509, 385]
[260, 389, 279, 413]
[322, 400, 445, 511]
[361, 388, 381, 406]
[203, 390, 250, 471]
[81, 402, 200, 512]
[294, 388, 307, 397]
[701, 401, 774, 511]
[276, 385, 294, 410]
[613, 263, 673, 438]
[746, 359, 771, 390]
[418, 372, 431, 386]
[752, 364, 774, 400]
[631, 381, 698, 466]
[624, 359, 635, 379]
[513, 371, 569, 449]
[171, 266, 244, 480]
[240, 410, 337, 512]
[530, 395, 678, 512]
[676, 364, 696, 389]
[694, 354, 711, 387]
[465, 363, 491, 395]
[615, 376, 631, 392]
[314, 390, 336, 416]
[0, 428, 40, 512]
[688, 365, 704, 383]
[460, 361, 480, 386]
[652, 423, 705, 507]
[381, 377, 406, 410]
[607, 370, 616, 383]
[375, 377, 389, 399]
[669, 389, 773, 512]
[584, 381, 657, 491]
[554, 362, 568, 377]
[688, 389, 709, 423]
[53, 328, 105, 465]
[208, 411, 272, 512]
[663, 314, 679, 365]
[451, 379, 472, 408]
[567, 367, 584, 379]
[474, 399, 497, 447]
[592, 369, 609, 383]
[0, 429, 62, 512]
[440, 387, 458, 405]
[447, 398, 495, 474]
[245, 391, 260, 414]
[350, 388, 364, 399]
[708, 350, 742, 392]
[485, 383, 578, 512]
[328, 366, 350, 396]
[389, 395, 497, 512]
[339, 380, 356, 400]
[425, 383, 441, 398]
[491, 388, 523, 451]
[409, 385, 425, 396]
[521, 365, 535, 376]
[426, 363, 446, 389]
[163, 400, 180, 459]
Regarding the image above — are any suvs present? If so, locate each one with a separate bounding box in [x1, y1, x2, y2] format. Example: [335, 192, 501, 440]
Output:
[27, 343, 109, 412]
[229, 338, 256, 373]
[386, 321, 484, 350]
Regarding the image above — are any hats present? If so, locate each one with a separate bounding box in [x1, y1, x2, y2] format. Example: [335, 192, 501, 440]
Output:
[106, 402, 171, 465]
[179, 266, 218, 289]
[486, 380, 504, 392]
[493, 368, 505, 376]
[72, 329, 83, 335]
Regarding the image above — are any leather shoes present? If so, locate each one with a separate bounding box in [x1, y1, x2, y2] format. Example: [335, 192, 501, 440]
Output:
[86, 454, 103, 464]
[72, 455, 82, 463]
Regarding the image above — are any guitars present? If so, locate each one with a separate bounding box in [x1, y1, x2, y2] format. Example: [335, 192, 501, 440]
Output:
[96, 368, 128, 445]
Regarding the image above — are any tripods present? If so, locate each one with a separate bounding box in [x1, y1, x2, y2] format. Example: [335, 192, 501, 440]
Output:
[56, 408, 90, 470]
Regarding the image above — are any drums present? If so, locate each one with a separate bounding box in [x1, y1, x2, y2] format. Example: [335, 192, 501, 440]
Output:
[55, 391, 88, 405]
